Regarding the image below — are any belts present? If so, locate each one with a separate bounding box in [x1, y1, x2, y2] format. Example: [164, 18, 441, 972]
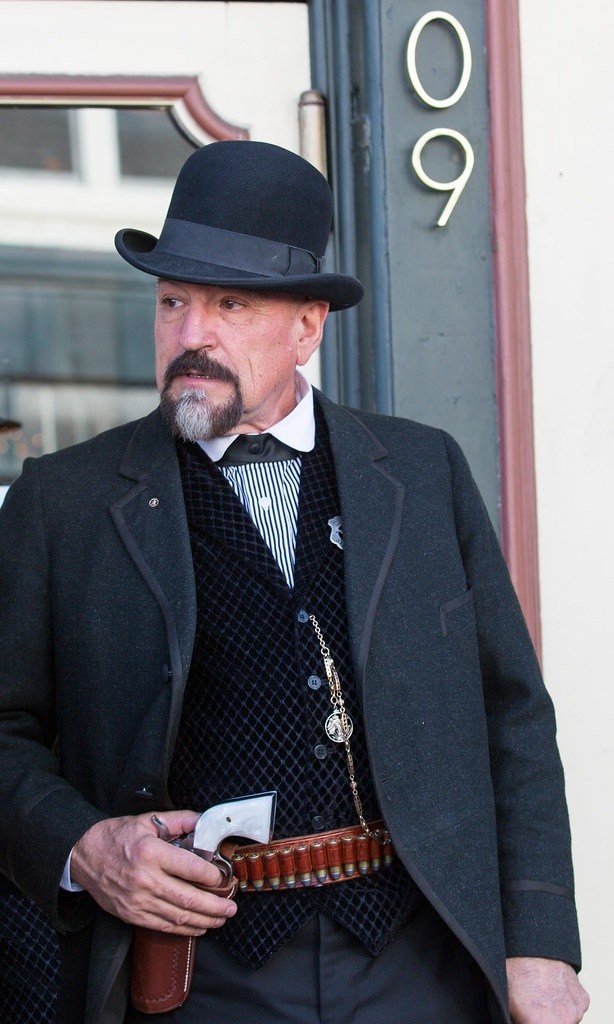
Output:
[226, 823, 397, 892]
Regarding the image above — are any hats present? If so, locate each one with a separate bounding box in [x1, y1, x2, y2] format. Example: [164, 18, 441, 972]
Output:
[115, 140, 364, 312]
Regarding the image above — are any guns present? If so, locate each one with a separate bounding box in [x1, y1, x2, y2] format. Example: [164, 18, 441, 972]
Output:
[152, 790, 280, 886]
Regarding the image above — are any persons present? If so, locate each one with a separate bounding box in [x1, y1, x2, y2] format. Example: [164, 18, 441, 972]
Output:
[0, 141, 589, 1023]
